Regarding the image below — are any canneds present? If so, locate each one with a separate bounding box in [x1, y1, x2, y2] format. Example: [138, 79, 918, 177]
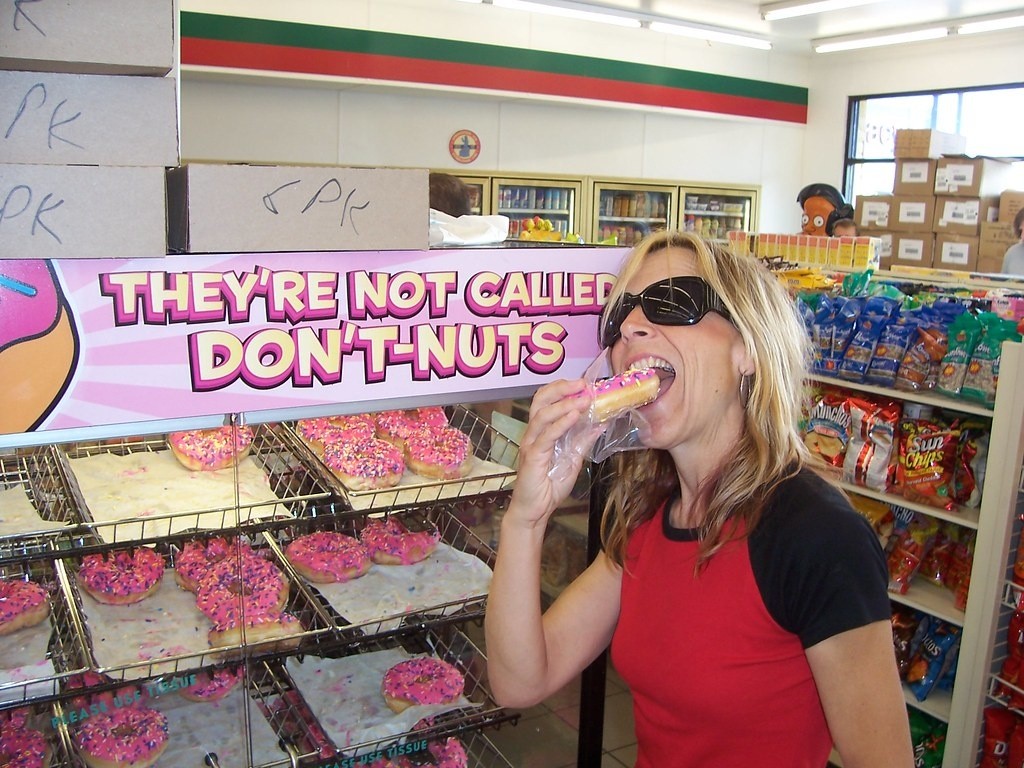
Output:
[600, 195, 659, 218]
[499, 186, 569, 239]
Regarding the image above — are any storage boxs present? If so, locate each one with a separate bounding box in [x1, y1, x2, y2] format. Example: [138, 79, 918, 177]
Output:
[851, 129, 1024, 273]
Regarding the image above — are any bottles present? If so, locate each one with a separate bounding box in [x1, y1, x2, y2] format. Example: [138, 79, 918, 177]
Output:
[682, 214, 742, 240]
[598, 223, 642, 248]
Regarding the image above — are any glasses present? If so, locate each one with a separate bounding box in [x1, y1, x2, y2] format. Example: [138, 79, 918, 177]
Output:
[597, 275, 737, 347]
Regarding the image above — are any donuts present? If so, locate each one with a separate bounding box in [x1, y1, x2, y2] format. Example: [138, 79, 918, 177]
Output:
[562, 366, 660, 422]
[0, 404, 473, 768]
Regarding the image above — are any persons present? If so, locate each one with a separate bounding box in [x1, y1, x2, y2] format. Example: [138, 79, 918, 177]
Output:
[1001, 207, 1024, 275]
[429, 172, 470, 218]
[485, 229, 913, 768]
[833, 220, 859, 237]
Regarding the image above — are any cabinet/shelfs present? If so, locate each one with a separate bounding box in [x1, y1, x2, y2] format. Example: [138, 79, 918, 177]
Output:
[781, 270, 1024, 768]
[588, 177, 764, 244]
[0, 398, 615, 768]
[422, 164, 585, 247]
